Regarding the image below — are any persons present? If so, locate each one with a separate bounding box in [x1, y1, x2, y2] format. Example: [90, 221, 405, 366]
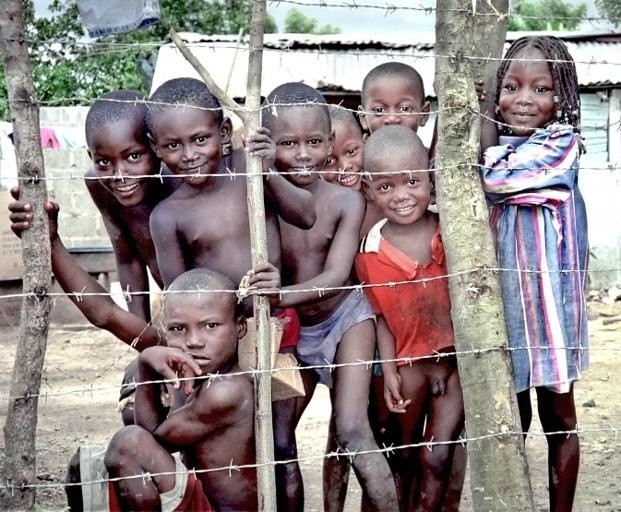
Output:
[8, 187, 259, 512]
[80, 62, 470, 512]
[477, 34, 589, 512]
